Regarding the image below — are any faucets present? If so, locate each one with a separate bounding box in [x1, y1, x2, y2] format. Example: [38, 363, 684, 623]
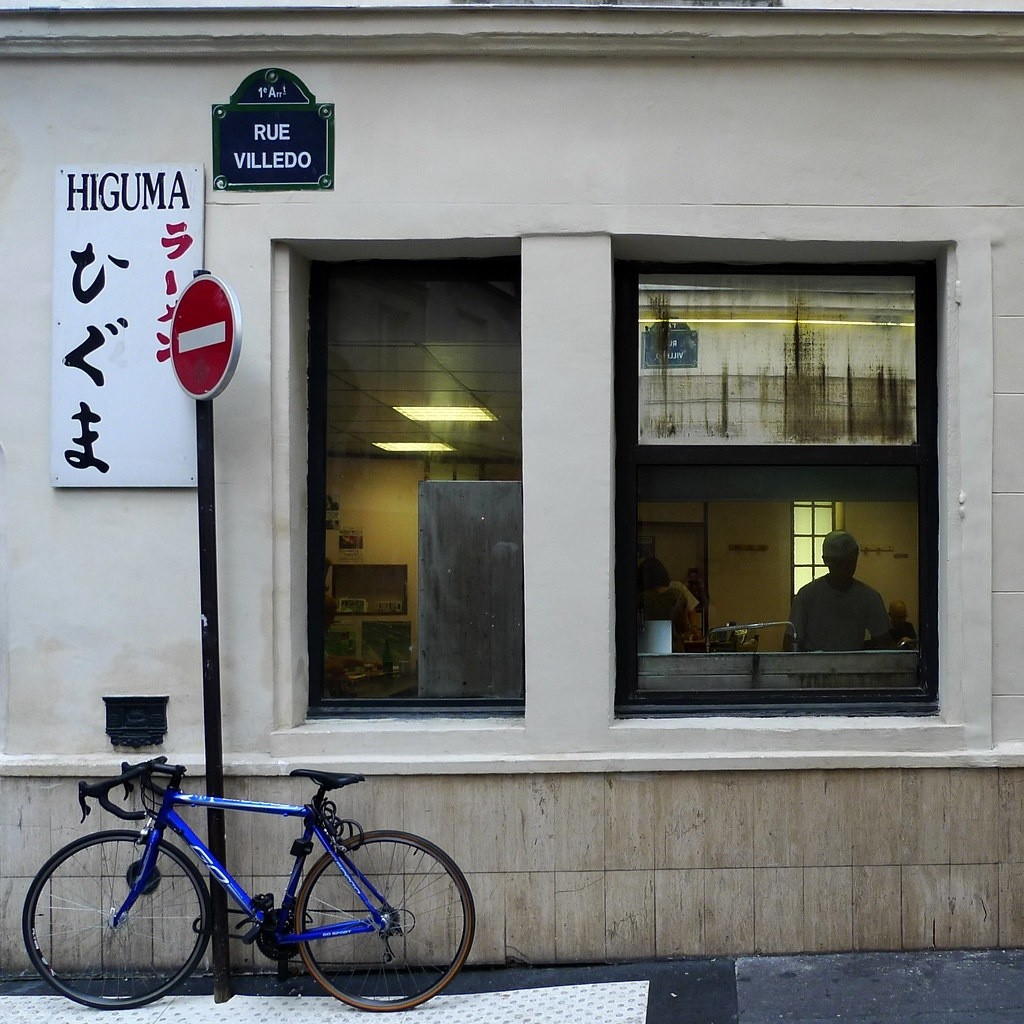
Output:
[764, 620, 800, 642]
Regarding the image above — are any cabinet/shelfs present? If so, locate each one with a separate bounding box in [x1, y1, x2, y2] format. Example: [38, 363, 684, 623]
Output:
[328, 616, 418, 676]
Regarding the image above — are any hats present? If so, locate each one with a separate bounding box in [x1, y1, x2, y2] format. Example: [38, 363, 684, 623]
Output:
[889, 601, 910, 618]
[823, 531, 859, 557]
[688, 568, 697, 576]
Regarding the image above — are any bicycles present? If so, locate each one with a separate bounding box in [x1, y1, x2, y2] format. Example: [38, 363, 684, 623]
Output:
[23, 759, 477, 1012]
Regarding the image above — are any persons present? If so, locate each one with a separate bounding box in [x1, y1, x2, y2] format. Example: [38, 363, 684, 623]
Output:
[324, 594, 364, 698]
[888, 600, 918, 648]
[638, 557, 700, 641]
[782, 529, 897, 651]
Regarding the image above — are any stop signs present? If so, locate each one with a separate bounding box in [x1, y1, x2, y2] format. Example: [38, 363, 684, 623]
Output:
[170, 275, 239, 401]
